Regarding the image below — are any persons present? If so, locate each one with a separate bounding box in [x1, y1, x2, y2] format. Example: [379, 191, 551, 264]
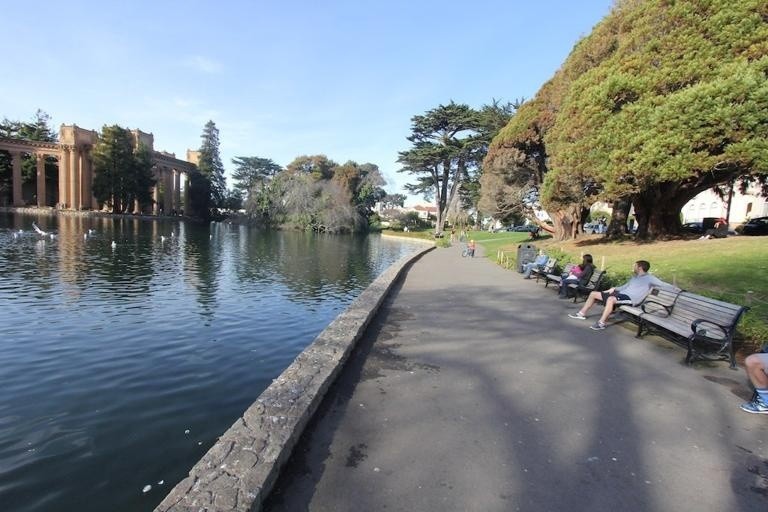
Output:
[739, 352, 768, 414]
[405, 225, 414, 232]
[696, 222, 727, 240]
[567, 260, 679, 331]
[559, 263, 585, 287]
[450, 224, 476, 262]
[597, 214, 607, 234]
[557, 254, 593, 300]
[522, 248, 549, 279]
[404, 225, 408, 232]
[528, 227, 541, 241]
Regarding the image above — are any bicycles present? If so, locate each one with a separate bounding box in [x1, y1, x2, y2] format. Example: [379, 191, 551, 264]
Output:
[461, 248, 471, 258]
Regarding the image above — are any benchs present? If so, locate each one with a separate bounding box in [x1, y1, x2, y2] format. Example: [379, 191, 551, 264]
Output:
[535, 258, 752, 370]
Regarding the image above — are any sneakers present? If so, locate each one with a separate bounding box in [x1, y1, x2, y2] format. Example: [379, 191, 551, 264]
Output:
[590, 321, 607, 330]
[740, 396, 768, 414]
[567, 312, 586, 320]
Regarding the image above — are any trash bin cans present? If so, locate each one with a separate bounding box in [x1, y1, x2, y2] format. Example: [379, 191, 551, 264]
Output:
[444, 221, 448, 228]
[703, 218, 719, 233]
[516, 243, 537, 273]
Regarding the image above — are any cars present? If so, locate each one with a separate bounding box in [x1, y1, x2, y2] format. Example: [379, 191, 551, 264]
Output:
[743, 217, 768, 235]
[583, 224, 608, 235]
[680, 222, 704, 234]
[507, 226, 537, 233]
